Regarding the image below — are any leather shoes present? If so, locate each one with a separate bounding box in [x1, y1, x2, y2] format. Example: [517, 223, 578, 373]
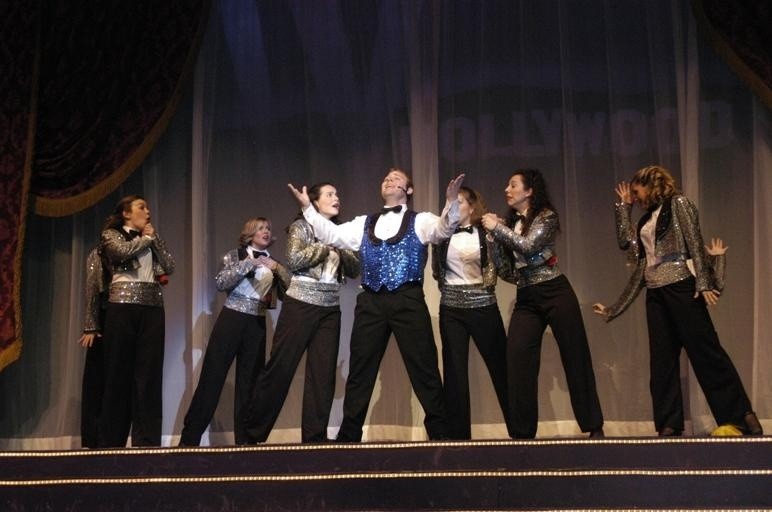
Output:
[590, 429, 605, 439]
[744, 411, 763, 435]
[659, 427, 682, 436]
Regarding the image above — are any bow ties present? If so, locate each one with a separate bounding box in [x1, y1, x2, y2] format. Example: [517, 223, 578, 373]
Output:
[380, 205, 402, 214]
[453, 226, 473, 233]
[129, 230, 142, 238]
[253, 250, 267, 259]
[515, 215, 525, 222]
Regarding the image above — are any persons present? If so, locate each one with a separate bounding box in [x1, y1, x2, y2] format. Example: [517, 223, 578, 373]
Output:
[180, 217, 293, 447]
[288, 169, 467, 444]
[240, 183, 361, 444]
[78, 215, 114, 453]
[614, 180, 729, 305]
[591, 164, 762, 438]
[430, 184, 518, 440]
[93, 196, 177, 449]
[480, 169, 606, 440]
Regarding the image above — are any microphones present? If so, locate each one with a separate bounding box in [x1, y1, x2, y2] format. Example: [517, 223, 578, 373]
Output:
[398, 186, 407, 193]
